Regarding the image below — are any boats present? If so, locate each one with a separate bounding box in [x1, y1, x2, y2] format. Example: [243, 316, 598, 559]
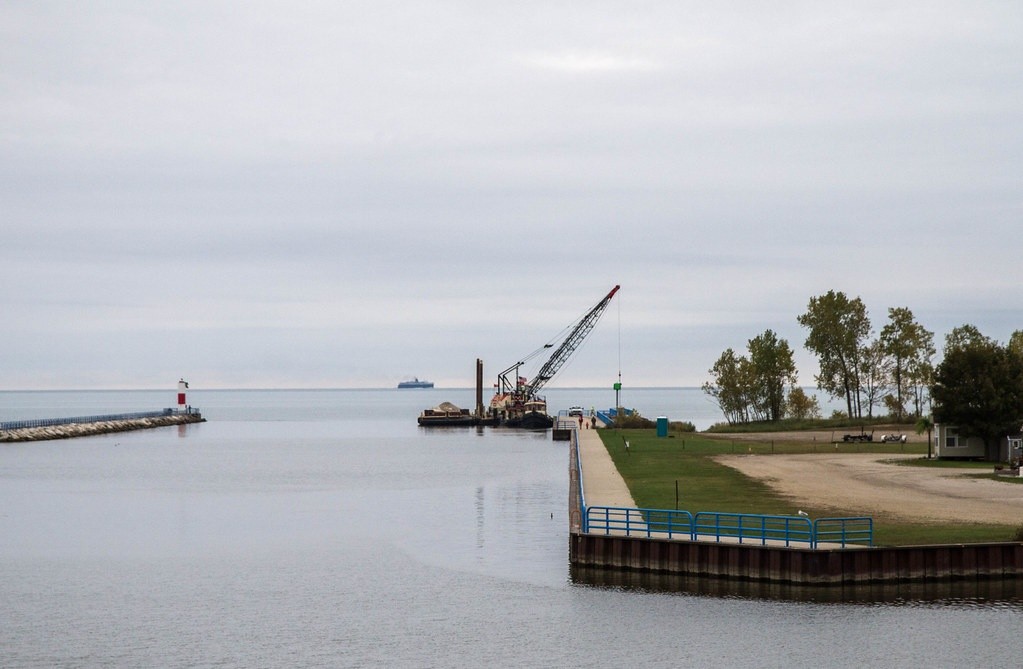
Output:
[398, 378, 434, 388]
[416, 409, 480, 426]
[486, 387, 553, 430]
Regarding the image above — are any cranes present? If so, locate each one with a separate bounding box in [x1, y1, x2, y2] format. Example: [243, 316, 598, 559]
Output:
[498, 284, 621, 410]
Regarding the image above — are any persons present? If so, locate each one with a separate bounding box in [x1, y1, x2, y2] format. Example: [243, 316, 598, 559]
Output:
[590, 406, 595, 416]
[586, 422, 589, 429]
[579, 417, 583, 429]
[591, 417, 596, 426]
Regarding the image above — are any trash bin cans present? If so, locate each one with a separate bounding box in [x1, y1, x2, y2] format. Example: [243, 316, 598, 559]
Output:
[657, 417, 668, 437]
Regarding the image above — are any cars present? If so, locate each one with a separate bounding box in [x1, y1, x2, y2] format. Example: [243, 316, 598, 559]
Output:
[881, 433, 908, 444]
[568, 405, 585, 417]
[841, 433, 874, 441]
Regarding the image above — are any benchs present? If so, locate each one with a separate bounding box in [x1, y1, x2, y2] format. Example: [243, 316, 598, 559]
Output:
[993, 465, 1020, 477]
